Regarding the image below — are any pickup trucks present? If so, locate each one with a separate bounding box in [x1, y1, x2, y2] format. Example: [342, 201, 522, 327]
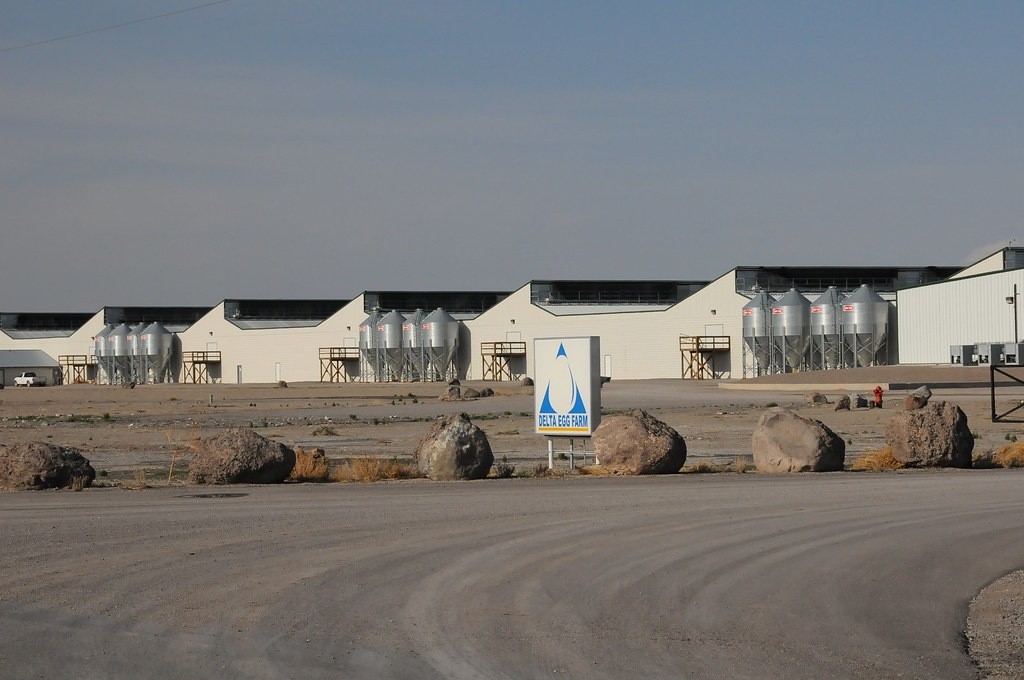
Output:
[14, 371, 47, 388]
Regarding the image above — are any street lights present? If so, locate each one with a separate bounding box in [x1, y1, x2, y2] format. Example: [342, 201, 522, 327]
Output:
[1005, 284, 1018, 343]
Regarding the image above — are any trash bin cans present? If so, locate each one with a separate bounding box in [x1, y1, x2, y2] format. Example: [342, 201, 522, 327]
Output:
[57, 376, 61, 385]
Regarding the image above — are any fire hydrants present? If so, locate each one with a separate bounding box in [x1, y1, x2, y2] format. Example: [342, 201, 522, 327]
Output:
[872, 386, 883, 408]
